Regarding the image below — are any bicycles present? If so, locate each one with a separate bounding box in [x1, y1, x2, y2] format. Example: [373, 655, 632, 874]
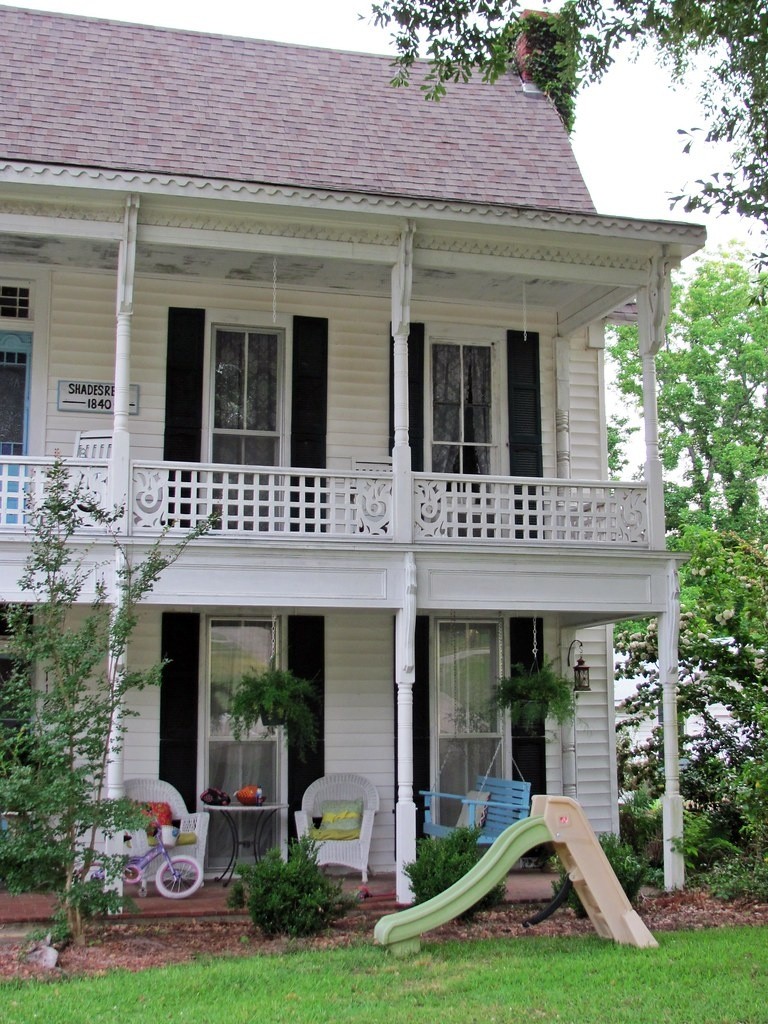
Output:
[67, 821, 203, 905]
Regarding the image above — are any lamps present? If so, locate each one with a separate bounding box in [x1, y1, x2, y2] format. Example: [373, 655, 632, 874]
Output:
[568, 639, 592, 692]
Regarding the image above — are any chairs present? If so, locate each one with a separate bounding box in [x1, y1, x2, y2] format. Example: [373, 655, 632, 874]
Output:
[295, 774, 380, 884]
[123, 778, 209, 898]
[352, 456, 392, 535]
[34, 430, 113, 528]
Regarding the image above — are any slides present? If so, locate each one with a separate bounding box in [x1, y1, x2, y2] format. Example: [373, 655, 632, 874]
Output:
[373, 814, 553, 958]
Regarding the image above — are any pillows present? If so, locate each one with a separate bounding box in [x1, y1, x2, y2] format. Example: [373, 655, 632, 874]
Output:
[127, 801, 172, 837]
[455, 789, 491, 828]
[319, 798, 363, 831]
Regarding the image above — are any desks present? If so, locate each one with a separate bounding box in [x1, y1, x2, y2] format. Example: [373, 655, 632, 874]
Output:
[202, 803, 289, 887]
